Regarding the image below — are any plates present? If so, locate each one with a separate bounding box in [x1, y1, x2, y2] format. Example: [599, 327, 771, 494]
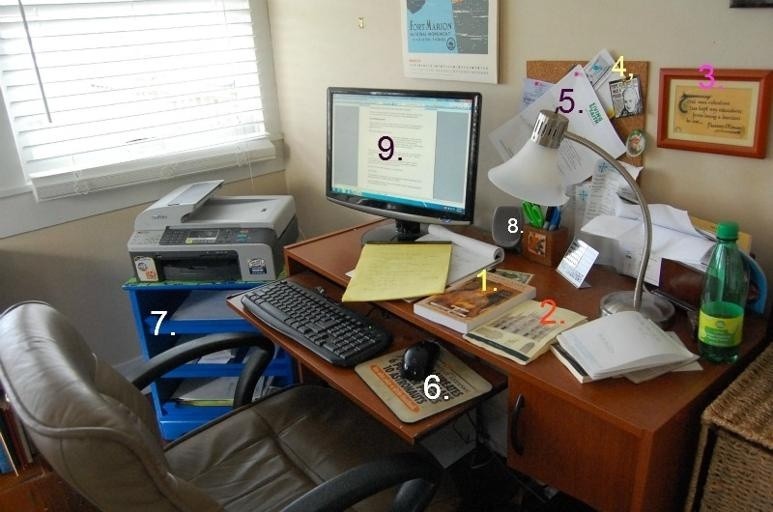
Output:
[0, 382, 98, 512]
[671, 343, 771, 512]
[123, 265, 300, 441]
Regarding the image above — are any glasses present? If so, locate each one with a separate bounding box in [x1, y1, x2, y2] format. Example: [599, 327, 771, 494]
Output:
[488, 109, 677, 330]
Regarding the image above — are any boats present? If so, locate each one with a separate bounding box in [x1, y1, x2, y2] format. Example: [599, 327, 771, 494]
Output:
[489, 204, 523, 251]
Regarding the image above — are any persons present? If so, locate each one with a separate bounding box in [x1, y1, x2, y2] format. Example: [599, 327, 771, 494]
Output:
[618, 85, 643, 118]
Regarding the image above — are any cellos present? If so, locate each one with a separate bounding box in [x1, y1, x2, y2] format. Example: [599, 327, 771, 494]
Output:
[124, 177, 303, 284]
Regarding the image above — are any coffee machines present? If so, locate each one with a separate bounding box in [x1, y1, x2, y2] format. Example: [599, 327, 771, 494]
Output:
[522, 202, 544, 228]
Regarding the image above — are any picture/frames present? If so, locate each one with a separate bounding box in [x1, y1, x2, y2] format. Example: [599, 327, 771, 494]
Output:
[227, 217, 772, 512]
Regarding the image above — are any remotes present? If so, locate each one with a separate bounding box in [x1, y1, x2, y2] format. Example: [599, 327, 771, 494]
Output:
[402, 335, 441, 380]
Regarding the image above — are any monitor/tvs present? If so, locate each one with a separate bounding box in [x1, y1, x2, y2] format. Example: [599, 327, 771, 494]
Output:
[170, 376, 274, 407]
[345, 224, 505, 304]
[557, 310, 694, 382]
[342, 240, 452, 303]
[0, 394, 37, 477]
[462, 299, 589, 365]
[548, 342, 596, 385]
[413, 272, 537, 335]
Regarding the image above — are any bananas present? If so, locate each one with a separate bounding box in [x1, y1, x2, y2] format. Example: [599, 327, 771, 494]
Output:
[240, 278, 393, 370]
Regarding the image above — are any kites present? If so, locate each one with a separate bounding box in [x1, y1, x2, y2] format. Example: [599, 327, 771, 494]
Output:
[543, 207, 556, 230]
[550, 210, 562, 232]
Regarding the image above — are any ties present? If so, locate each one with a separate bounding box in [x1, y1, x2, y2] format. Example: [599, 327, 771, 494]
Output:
[324, 86, 483, 248]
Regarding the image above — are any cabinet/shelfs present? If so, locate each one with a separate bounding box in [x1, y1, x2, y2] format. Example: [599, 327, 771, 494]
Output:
[697, 221, 746, 365]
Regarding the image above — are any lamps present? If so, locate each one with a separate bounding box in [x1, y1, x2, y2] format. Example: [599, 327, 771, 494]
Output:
[1, 300, 442, 512]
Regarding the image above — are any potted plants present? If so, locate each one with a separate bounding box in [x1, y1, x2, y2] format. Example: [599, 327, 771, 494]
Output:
[658, 68, 771, 160]
